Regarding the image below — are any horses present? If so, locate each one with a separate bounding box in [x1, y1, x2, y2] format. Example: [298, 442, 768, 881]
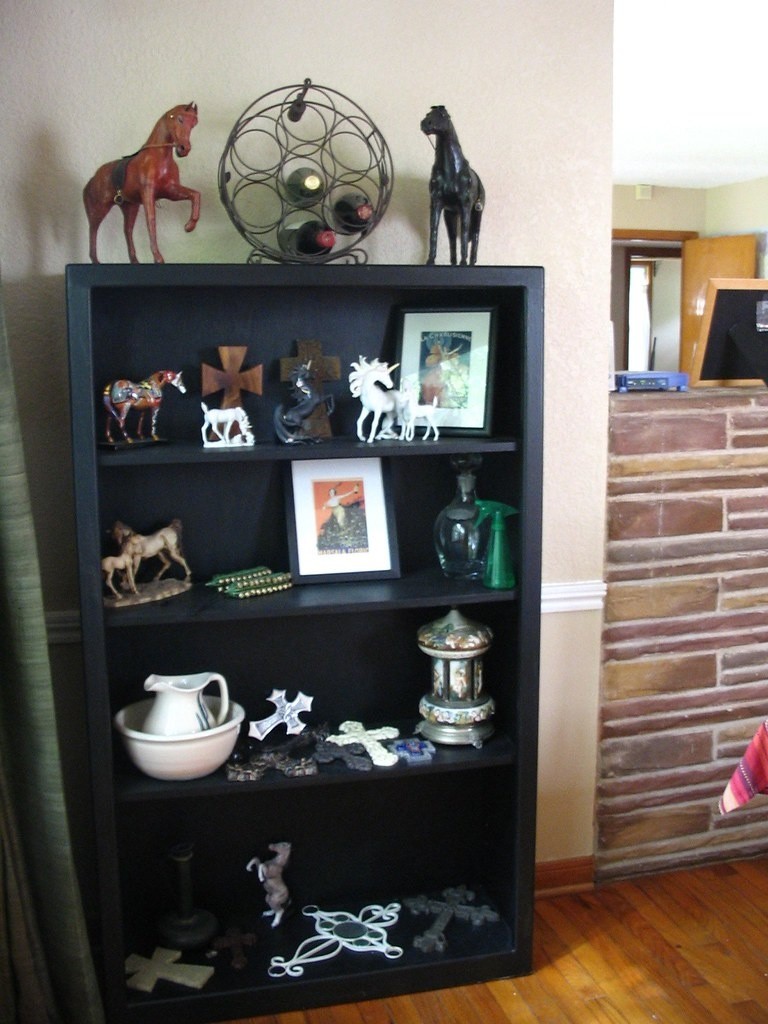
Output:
[246, 839, 293, 928]
[82, 101, 202, 264]
[272, 359, 335, 445]
[397, 386, 440, 441]
[103, 370, 187, 444]
[201, 400, 253, 442]
[102, 516, 191, 600]
[348, 356, 406, 443]
[420, 105, 485, 266]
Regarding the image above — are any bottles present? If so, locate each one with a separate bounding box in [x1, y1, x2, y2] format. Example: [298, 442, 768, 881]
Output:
[434, 472, 490, 585]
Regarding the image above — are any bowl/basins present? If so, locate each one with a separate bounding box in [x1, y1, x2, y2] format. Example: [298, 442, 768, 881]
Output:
[113, 697, 245, 782]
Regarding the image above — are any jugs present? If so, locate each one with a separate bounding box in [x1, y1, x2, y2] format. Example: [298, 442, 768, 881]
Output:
[143, 672, 229, 735]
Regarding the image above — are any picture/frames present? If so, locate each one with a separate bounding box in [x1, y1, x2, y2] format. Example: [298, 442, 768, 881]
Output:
[283, 460, 401, 584]
[389, 304, 498, 438]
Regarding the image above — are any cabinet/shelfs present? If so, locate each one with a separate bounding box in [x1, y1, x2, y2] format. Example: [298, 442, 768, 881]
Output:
[64, 264, 545, 1023]
